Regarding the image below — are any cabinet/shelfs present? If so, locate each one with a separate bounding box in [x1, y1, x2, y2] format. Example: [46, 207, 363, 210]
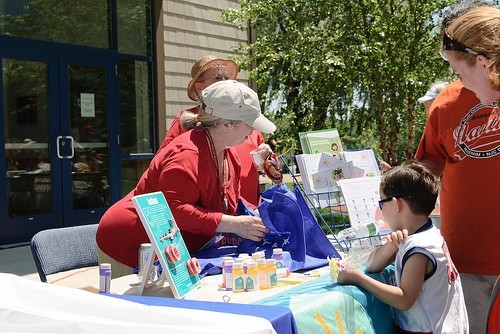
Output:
[281, 147, 398, 252]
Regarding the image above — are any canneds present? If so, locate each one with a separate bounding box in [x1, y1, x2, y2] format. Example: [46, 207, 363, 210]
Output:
[139, 243, 163, 287]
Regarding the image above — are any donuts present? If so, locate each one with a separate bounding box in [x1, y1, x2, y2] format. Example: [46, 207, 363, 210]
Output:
[186, 257, 201, 275]
[165, 245, 181, 264]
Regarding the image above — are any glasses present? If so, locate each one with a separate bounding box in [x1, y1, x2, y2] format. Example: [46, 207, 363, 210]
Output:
[378, 192, 409, 211]
[204, 76, 228, 86]
[441, 28, 489, 59]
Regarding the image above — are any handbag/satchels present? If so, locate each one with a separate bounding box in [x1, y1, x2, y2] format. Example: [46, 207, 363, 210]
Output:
[236, 185, 341, 272]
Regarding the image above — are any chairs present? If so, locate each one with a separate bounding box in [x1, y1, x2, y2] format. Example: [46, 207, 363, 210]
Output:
[29, 223, 100, 282]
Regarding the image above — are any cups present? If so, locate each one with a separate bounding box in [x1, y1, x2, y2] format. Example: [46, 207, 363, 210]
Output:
[249, 149, 265, 171]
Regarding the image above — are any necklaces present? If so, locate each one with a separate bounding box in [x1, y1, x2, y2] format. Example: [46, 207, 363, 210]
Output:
[204, 127, 229, 210]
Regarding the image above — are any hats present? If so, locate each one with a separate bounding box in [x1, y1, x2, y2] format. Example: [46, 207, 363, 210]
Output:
[186, 54, 237, 102]
[199, 80, 277, 134]
[418, 91, 438, 102]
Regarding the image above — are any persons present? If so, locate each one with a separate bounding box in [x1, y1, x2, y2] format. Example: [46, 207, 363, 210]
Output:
[96, 80, 272, 278]
[336, 5, 500, 334]
[156, 56, 282, 207]
[7, 127, 110, 201]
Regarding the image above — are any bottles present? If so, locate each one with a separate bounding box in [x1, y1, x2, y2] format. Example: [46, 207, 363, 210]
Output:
[139, 244, 153, 284]
[336, 219, 385, 248]
[222, 249, 286, 293]
[99, 262, 111, 295]
